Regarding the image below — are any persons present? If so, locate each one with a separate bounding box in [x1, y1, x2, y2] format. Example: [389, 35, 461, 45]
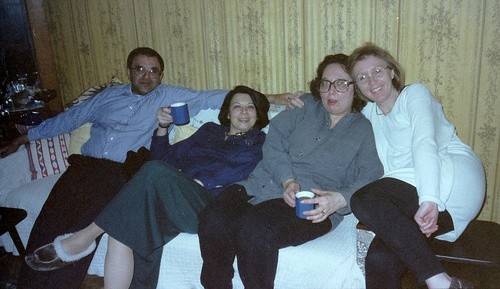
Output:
[289, 44, 486, 289]
[197, 54, 385, 289]
[23, 84, 270, 289]
[0, 46, 292, 289]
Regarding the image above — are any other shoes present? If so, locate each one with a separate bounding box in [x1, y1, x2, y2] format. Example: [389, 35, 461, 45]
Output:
[448, 276, 473, 289]
[24, 232, 96, 271]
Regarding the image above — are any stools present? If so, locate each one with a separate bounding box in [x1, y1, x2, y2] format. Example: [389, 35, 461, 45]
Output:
[429, 220, 500, 289]
[0, 207, 27, 257]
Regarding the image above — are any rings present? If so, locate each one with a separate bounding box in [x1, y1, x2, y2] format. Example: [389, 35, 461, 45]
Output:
[321, 211, 326, 216]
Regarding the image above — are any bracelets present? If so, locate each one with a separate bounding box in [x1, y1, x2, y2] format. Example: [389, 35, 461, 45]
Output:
[158, 122, 170, 129]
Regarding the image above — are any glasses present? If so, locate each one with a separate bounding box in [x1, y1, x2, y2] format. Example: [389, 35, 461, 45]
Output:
[315, 79, 356, 94]
[354, 65, 390, 86]
[128, 65, 162, 79]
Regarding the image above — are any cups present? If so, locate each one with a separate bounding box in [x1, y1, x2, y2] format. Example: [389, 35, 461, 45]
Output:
[295, 190, 316, 220]
[167, 102, 190, 126]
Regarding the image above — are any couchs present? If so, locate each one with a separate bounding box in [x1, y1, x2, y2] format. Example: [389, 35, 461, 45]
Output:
[0, 82, 376, 289]
